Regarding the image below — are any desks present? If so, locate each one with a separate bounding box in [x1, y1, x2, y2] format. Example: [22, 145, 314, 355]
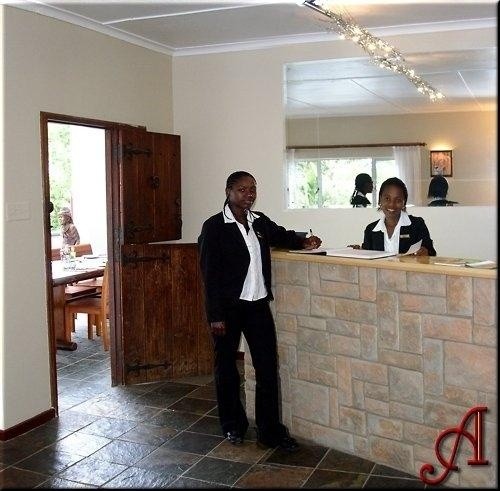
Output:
[52, 255, 106, 351]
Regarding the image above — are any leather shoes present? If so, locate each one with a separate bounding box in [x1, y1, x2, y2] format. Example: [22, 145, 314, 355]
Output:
[258, 432, 298, 450]
[224, 429, 244, 444]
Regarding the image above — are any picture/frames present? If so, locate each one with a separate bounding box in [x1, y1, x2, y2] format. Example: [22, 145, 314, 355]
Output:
[429, 150, 453, 179]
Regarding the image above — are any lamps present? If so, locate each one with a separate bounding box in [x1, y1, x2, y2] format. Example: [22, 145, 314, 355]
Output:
[373, 49, 447, 107]
[297, 2, 399, 58]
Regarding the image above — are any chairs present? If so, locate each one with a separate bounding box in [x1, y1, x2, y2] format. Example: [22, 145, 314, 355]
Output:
[50, 248, 99, 304]
[72, 245, 103, 289]
[64, 263, 110, 352]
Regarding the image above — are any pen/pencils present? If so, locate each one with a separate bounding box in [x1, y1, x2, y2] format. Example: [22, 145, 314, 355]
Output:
[310, 228, 313, 236]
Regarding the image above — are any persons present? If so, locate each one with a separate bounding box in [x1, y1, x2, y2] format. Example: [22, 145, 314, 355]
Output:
[347, 177, 436, 256]
[427, 175, 458, 206]
[350, 173, 373, 206]
[197, 170, 322, 451]
[58, 207, 80, 260]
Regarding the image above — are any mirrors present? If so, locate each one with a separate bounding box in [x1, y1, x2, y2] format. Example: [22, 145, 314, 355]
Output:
[281, 47, 498, 208]
